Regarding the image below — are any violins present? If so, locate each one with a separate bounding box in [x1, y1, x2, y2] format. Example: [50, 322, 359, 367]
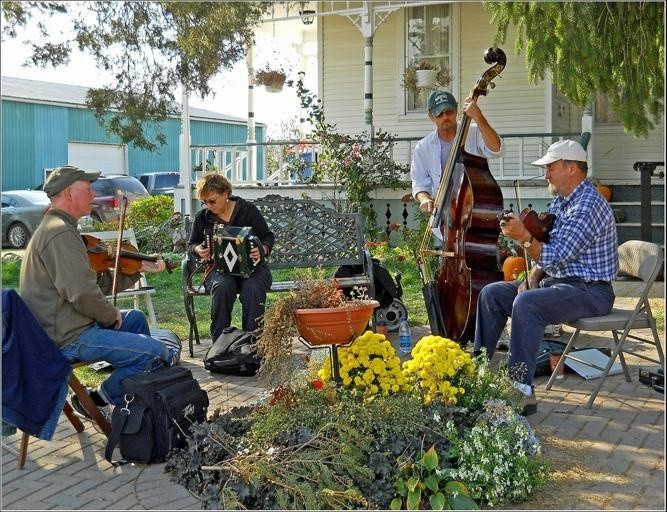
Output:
[79, 234, 178, 275]
[498, 206, 556, 247]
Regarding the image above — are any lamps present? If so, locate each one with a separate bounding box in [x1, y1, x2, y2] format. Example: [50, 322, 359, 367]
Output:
[299, 8, 316, 26]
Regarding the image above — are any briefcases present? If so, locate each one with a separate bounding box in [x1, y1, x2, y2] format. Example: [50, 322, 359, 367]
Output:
[639, 365, 664, 394]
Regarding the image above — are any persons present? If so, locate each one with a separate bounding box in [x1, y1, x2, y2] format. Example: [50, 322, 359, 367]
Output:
[20, 164, 175, 435]
[410, 89, 512, 351]
[465, 138, 622, 418]
[184, 173, 275, 346]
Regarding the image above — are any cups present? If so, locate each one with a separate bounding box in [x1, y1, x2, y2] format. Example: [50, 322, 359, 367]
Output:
[549, 351, 565, 379]
[375, 321, 388, 338]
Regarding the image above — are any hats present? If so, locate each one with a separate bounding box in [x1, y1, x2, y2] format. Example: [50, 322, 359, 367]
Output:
[530, 139, 588, 167]
[426, 88, 458, 118]
[40, 165, 103, 199]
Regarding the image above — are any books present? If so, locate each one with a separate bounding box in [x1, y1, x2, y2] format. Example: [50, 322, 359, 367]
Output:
[560, 349, 624, 381]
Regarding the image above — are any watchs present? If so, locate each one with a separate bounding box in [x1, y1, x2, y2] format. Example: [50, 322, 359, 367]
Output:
[519, 235, 534, 249]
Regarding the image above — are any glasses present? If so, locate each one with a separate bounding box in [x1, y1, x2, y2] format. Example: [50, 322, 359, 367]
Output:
[200, 199, 218, 207]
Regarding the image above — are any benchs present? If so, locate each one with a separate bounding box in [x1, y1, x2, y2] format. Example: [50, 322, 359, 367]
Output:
[182, 192, 375, 360]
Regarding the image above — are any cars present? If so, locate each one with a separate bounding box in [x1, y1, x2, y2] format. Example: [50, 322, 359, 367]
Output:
[0, 187, 97, 250]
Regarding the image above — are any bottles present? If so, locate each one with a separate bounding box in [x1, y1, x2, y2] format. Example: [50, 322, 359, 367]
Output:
[398, 316, 411, 353]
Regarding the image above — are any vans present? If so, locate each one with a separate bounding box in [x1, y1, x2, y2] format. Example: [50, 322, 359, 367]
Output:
[29, 172, 156, 227]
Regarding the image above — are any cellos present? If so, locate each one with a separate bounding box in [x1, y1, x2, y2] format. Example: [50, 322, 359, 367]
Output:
[419, 48, 506, 348]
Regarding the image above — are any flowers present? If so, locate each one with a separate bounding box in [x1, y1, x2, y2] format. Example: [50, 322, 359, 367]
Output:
[248, 258, 374, 388]
[399, 60, 453, 96]
[247, 61, 288, 89]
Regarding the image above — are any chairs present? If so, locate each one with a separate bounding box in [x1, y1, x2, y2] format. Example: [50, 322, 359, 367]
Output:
[76, 226, 157, 331]
[543, 235, 662, 410]
[0, 285, 113, 470]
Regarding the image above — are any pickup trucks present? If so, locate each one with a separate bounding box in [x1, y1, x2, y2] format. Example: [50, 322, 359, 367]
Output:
[132, 170, 197, 198]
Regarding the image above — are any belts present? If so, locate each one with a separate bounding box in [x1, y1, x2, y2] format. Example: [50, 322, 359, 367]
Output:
[585, 279, 612, 286]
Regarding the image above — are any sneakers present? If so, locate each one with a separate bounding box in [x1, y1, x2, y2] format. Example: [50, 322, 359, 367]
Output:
[70, 387, 114, 434]
[498, 383, 539, 417]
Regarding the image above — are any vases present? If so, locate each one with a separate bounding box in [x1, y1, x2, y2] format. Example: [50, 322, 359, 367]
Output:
[414, 69, 436, 88]
[291, 297, 380, 345]
[265, 79, 284, 92]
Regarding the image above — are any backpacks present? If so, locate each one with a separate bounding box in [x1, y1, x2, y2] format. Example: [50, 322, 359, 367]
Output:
[203, 325, 266, 378]
[105, 357, 210, 468]
[333, 250, 395, 313]
